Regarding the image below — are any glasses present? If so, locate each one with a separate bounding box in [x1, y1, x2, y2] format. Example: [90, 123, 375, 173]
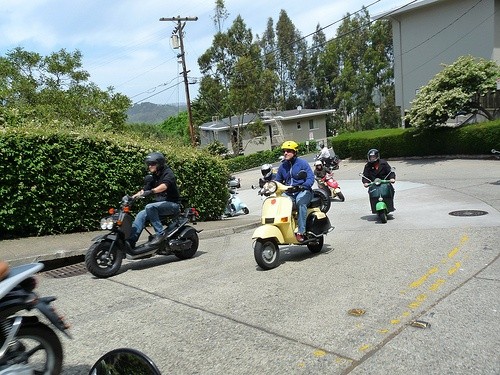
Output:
[147, 162, 157, 166]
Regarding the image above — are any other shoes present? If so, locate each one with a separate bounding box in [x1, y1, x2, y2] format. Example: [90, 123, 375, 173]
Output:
[296, 233, 304, 242]
[149, 235, 167, 246]
[126, 239, 136, 250]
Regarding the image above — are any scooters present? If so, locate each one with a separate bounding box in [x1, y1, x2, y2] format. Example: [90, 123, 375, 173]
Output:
[228, 176, 241, 189]
[86, 175, 204, 278]
[252, 170, 331, 270]
[314, 154, 339, 172]
[315, 168, 345, 202]
[227, 189, 250, 216]
[0, 262, 74, 375]
[358, 167, 395, 224]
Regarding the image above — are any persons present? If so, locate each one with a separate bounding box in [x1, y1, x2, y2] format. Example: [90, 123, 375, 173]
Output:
[270, 141, 315, 243]
[328, 145, 336, 159]
[277, 155, 286, 163]
[312, 161, 332, 197]
[317, 141, 330, 166]
[362, 149, 396, 214]
[259, 164, 277, 188]
[130, 153, 181, 248]
[0, 261, 11, 284]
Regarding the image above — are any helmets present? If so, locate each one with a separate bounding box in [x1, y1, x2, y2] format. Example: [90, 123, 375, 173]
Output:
[278, 156, 285, 162]
[320, 143, 324, 148]
[145, 151, 165, 170]
[367, 149, 380, 164]
[281, 141, 299, 152]
[261, 164, 273, 178]
[315, 161, 322, 166]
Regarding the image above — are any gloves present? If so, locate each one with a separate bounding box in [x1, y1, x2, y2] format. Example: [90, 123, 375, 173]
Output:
[297, 185, 305, 192]
[142, 190, 153, 199]
